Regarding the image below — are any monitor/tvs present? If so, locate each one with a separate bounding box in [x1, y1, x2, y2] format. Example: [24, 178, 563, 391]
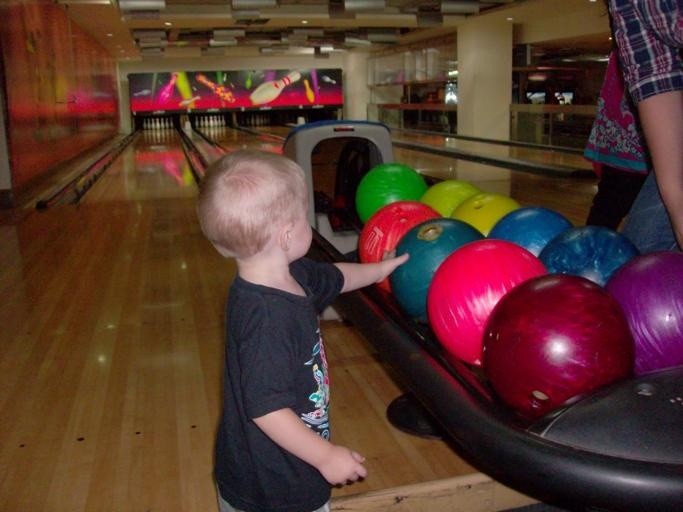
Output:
[554, 112, 575, 122]
[525, 90, 547, 104]
[554, 90, 575, 105]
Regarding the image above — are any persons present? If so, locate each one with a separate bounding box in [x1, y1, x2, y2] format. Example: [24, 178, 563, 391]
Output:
[196, 150, 410, 512]
[605, 0, 683, 254]
[586, 46, 653, 231]
